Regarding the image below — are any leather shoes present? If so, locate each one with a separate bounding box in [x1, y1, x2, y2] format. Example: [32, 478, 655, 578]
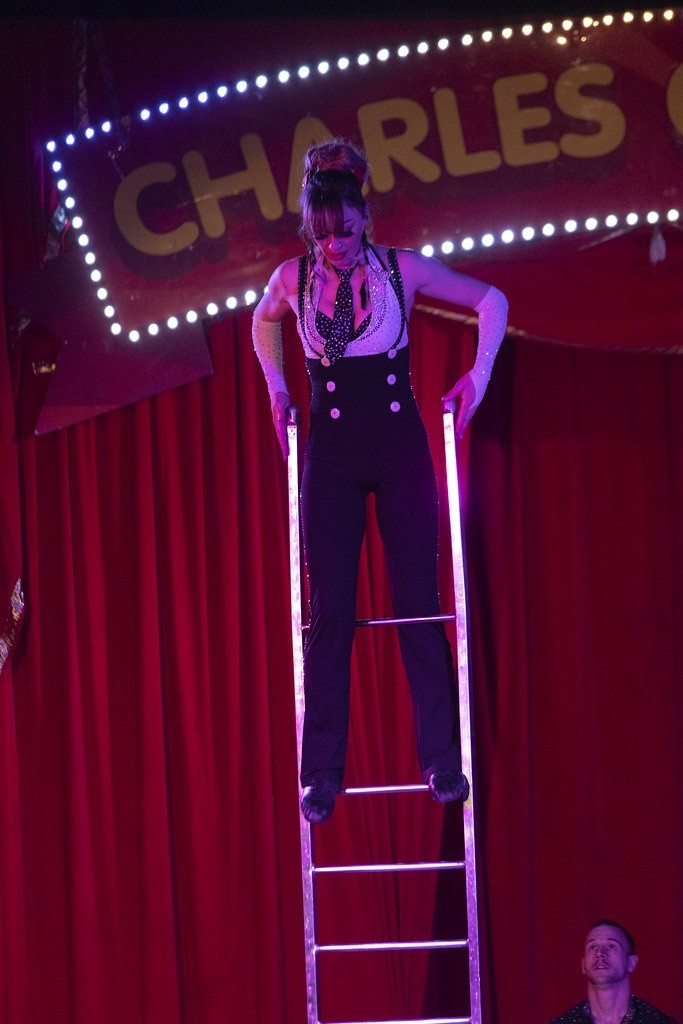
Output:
[425, 764, 467, 805]
[301, 782, 336, 823]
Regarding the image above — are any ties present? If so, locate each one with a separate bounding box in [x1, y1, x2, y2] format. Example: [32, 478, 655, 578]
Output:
[323, 265, 353, 365]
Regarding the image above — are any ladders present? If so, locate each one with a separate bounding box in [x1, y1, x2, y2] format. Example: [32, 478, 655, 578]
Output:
[284, 399, 485, 1023]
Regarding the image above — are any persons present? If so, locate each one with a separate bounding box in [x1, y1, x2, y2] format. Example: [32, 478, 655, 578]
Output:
[252, 141, 509, 825]
[546, 921, 680, 1024]
